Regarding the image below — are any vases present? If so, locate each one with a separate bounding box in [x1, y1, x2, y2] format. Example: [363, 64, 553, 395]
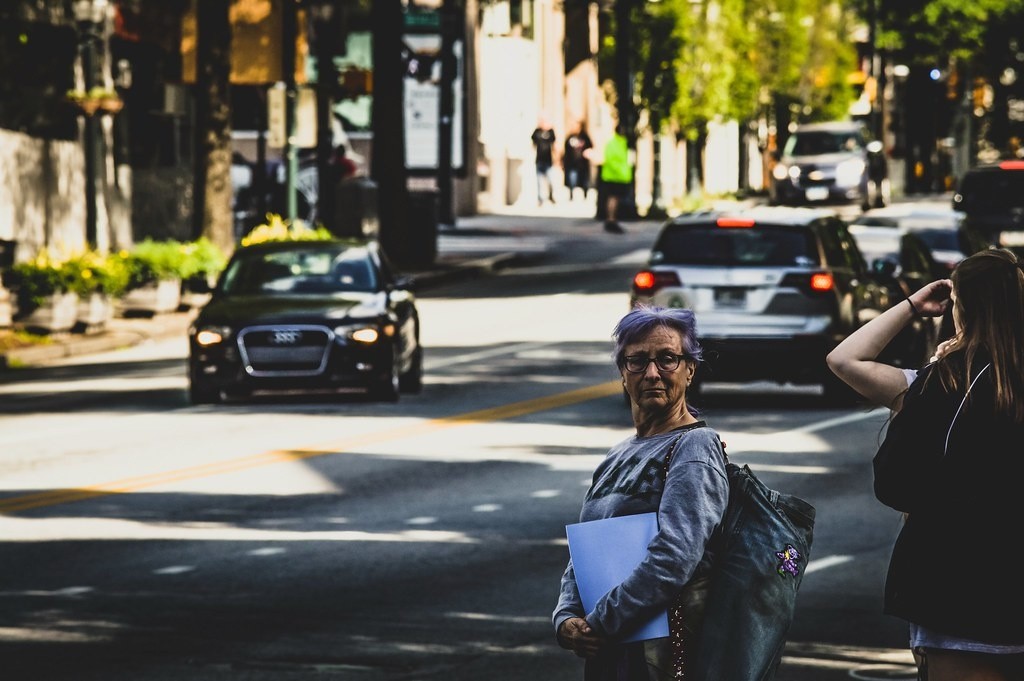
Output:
[27, 279, 183, 330]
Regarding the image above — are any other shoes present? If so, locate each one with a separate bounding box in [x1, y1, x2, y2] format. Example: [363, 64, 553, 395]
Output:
[605, 220, 624, 233]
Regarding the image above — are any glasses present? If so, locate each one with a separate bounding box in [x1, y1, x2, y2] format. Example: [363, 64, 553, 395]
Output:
[623, 353, 688, 372]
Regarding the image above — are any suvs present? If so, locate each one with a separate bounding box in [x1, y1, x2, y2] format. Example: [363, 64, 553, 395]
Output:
[767, 119, 883, 206]
[629, 203, 904, 403]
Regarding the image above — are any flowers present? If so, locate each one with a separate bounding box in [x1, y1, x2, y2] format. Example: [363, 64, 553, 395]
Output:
[2, 236, 231, 318]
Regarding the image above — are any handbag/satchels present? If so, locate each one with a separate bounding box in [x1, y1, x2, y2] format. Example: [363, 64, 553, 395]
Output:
[658, 427, 816, 681]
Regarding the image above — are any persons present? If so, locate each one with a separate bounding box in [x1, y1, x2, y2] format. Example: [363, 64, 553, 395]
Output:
[532, 111, 632, 235]
[824, 247, 1024, 680]
[551, 300, 729, 681]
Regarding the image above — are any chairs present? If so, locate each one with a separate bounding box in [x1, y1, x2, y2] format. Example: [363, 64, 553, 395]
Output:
[332, 258, 371, 288]
[248, 263, 292, 295]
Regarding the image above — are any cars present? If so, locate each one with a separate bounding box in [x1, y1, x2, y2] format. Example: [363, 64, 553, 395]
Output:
[853, 160, 1024, 298]
[184, 238, 424, 402]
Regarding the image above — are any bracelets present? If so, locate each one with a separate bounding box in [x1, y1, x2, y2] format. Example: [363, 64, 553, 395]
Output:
[907, 298, 919, 315]
